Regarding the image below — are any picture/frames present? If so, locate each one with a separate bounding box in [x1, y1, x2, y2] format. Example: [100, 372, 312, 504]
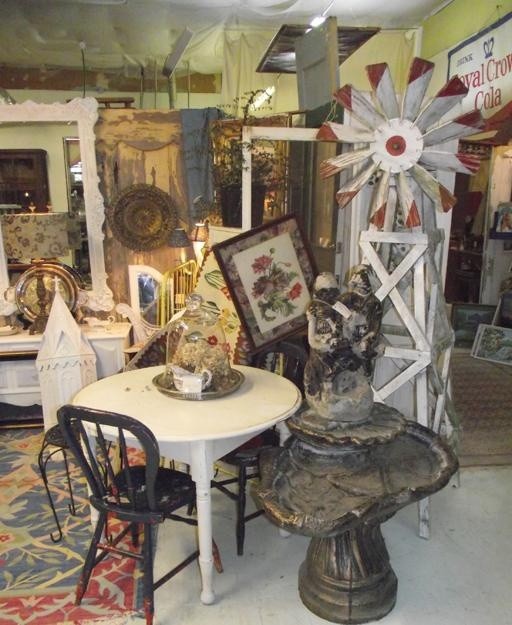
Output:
[205, 112, 291, 230]
[449, 303, 512, 367]
[211, 208, 322, 358]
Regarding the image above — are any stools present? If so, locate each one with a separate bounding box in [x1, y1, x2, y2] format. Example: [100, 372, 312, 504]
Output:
[36, 420, 84, 542]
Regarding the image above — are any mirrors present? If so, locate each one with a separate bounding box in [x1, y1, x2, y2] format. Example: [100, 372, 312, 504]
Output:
[0, 95, 116, 314]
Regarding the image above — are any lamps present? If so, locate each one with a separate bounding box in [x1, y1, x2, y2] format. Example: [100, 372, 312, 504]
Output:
[2, 210, 72, 335]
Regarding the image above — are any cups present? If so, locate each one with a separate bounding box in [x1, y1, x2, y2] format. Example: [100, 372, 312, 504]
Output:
[166, 363, 212, 393]
[83, 315, 116, 334]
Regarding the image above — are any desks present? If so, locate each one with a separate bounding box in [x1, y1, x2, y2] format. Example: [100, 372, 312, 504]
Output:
[71, 364, 302, 606]
[0, 323, 134, 419]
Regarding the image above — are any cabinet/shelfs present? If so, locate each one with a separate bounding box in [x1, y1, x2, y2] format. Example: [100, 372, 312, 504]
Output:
[1, 135, 89, 272]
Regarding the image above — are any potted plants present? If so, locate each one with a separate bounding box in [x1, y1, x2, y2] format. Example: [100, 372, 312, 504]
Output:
[210, 87, 304, 229]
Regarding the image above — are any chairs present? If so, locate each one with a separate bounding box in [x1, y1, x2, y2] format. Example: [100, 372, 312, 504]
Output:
[56, 404, 223, 624]
[187, 341, 308, 556]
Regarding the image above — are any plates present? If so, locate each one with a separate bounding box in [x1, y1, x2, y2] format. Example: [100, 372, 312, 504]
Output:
[0, 324, 21, 336]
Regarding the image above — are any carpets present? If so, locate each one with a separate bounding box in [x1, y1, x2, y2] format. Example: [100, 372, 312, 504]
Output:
[450, 352, 510, 471]
[0, 425, 159, 624]
